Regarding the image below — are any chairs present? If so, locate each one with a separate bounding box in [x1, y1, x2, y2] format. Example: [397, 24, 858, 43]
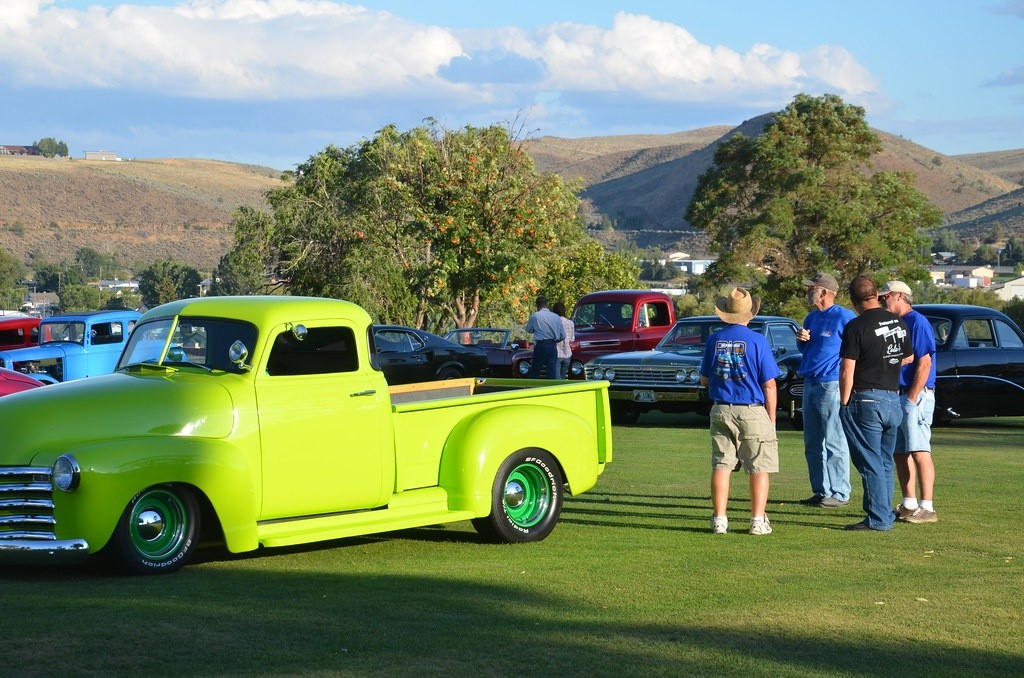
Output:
[477, 339, 492, 348]
[513, 340, 529, 349]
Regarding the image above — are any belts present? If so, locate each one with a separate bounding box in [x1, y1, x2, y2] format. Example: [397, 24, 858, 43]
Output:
[859, 388, 898, 393]
[924, 387, 934, 391]
[713, 401, 763, 407]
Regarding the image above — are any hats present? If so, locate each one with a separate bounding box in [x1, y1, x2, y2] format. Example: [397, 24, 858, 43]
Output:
[715, 288, 761, 325]
[803, 273, 839, 291]
[878, 280, 912, 296]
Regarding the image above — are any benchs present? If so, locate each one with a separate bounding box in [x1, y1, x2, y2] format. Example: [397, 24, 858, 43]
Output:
[93, 335, 122, 343]
[268, 352, 356, 374]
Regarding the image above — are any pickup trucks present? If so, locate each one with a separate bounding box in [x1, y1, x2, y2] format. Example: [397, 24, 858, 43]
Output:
[0, 294, 613, 569]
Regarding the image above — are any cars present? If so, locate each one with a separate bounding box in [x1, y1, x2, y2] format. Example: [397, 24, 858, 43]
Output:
[509, 290, 805, 422]
[785, 303, 1024, 424]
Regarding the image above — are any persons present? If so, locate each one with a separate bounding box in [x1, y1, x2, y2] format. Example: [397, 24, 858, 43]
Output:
[552, 302, 575, 380]
[797, 274, 937, 531]
[526, 296, 567, 380]
[698, 288, 780, 537]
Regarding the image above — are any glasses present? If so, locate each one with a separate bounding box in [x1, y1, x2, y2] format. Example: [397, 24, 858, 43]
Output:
[882, 296, 889, 300]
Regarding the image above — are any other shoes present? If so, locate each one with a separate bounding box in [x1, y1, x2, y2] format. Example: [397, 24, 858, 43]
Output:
[854, 520, 869, 529]
[800, 495, 825, 504]
[820, 498, 851, 507]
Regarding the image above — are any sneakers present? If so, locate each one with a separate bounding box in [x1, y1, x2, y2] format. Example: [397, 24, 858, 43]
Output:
[710, 516, 727, 534]
[892, 503, 917, 519]
[749, 514, 772, 535]
[904, 506, 938, 523]
